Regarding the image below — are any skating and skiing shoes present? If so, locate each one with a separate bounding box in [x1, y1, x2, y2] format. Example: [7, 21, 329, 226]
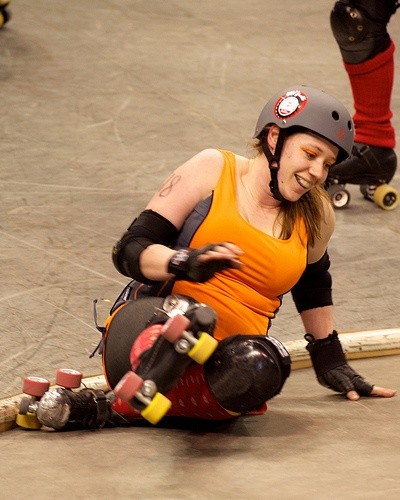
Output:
[15, 369, 109, 433]
[111, 304, 219, 425]
[325, 134, 399, 211]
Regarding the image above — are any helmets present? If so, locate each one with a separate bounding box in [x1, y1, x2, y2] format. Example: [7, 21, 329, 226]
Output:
[252, 86, 353, 167]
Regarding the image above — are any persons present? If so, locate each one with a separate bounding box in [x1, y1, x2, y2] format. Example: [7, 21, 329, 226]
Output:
[323, 0, 400, 186]
[36, 84, 398, 433]
